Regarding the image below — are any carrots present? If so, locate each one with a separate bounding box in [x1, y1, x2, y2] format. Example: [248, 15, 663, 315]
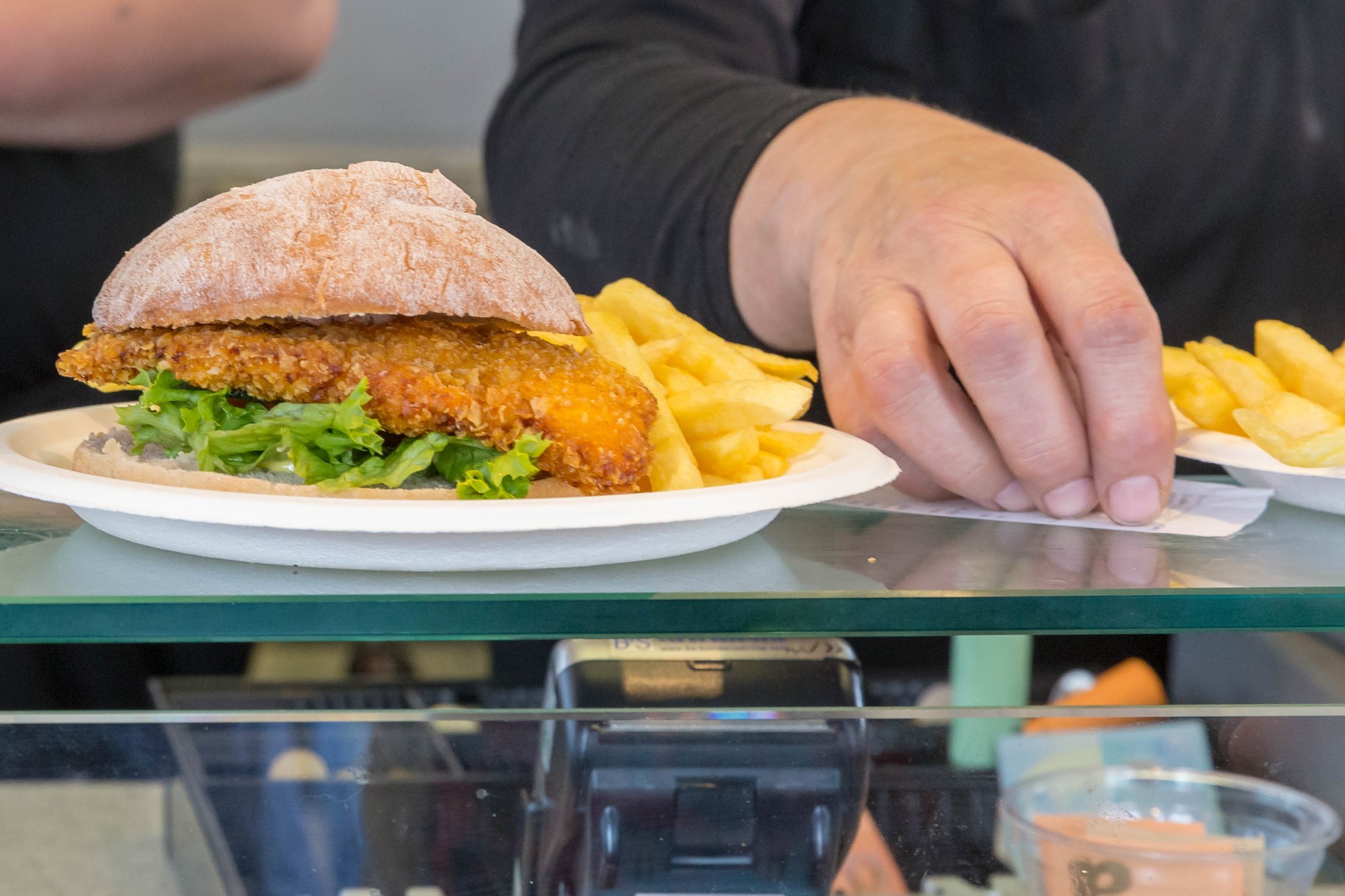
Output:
[1025, 658, 1164, 731]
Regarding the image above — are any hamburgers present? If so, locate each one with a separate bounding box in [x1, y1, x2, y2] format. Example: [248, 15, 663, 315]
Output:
[54, 160, 658, 499]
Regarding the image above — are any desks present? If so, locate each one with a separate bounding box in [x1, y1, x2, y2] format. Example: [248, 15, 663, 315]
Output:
[0, 482, 1345, 895]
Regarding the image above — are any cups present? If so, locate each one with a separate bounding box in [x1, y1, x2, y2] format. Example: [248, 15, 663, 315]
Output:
[997, 766, 1345, 896]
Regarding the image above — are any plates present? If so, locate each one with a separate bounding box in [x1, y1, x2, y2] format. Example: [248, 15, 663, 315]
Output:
[2, 402, 903, 573]
[1171, 399, 1345, 516]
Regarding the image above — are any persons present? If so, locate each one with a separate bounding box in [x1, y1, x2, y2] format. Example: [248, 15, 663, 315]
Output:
[0, 0, 340, 781]
[483, 0, 1345, 722]
[835, 510, 1168, 592]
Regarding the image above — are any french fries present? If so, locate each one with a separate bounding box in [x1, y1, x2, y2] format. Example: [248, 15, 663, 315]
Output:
[1161, 321, 1345, 469]
[518, 276, 828, 492]
